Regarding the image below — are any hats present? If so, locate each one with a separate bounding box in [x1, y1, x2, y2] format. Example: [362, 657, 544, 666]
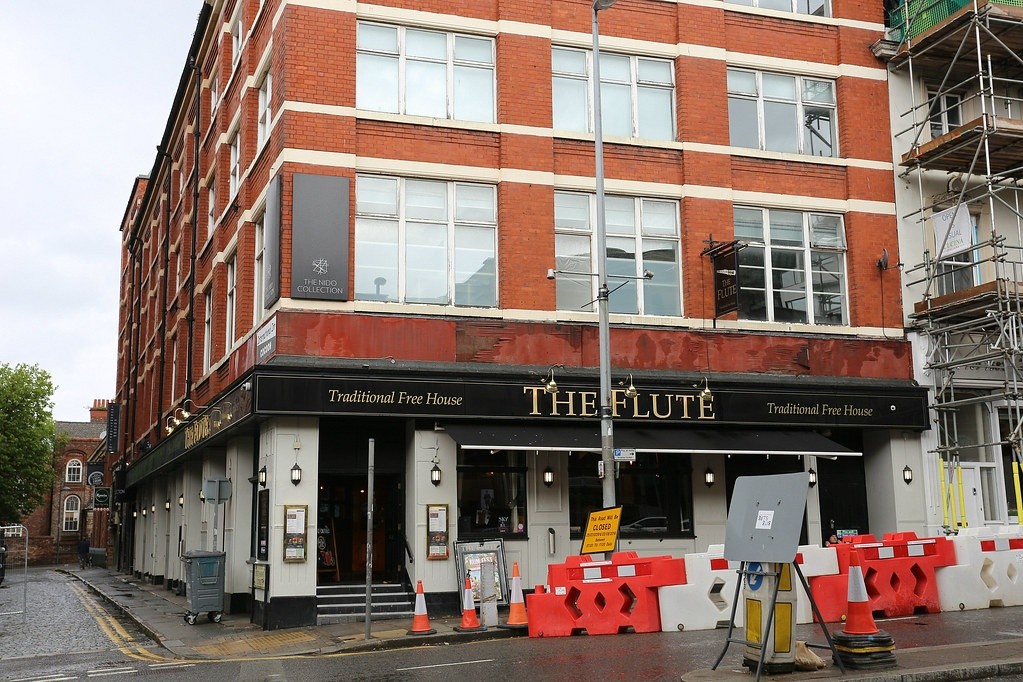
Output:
[827, 528, 836, 536]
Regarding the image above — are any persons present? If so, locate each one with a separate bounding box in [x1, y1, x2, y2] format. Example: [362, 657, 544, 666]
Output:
[825, 532, 842, 547]
[78, 535, 91, 571]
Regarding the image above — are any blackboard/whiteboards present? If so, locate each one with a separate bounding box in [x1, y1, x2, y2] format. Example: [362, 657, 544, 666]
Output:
[317, 518, 339, 572]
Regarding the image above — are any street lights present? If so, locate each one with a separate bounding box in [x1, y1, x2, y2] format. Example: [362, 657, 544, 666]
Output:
[55, 479, 71, 565]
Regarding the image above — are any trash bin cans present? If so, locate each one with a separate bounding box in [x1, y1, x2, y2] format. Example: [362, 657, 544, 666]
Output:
[89, 547, 106, 569]
[180, 551, 226, 625]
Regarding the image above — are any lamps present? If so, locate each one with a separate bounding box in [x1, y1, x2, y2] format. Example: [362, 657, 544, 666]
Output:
[539, 367, 558, 394]
[807, 468, 816, 487]
[618, 374, 639, 398]
[173, 408, 196, 426]
[165, 498, 170, 511]
[691, 377, 712, 401]
[133, 510, 137, 519]
[430, 464, 442, 487]
[199, 490, 204, 503]
[151, 504, 155, 514]
[904, 467, 913, 485]
[180, 398, 209, 420]
[705, 467, 715, 489]
[142, 506, 147, 518]
[165, 417, 189, 437]
[179, 494, 183, 508]
[291, 462, 301, 486]
[259, 465, 266, 487]
[543, 466, 555, 488]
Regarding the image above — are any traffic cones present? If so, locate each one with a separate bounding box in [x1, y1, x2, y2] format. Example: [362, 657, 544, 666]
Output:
[495, 562, 528, 630]
[453, 577, 488, 633]
[831, 546, 899, 670]
[405, 581, 438, 637]
